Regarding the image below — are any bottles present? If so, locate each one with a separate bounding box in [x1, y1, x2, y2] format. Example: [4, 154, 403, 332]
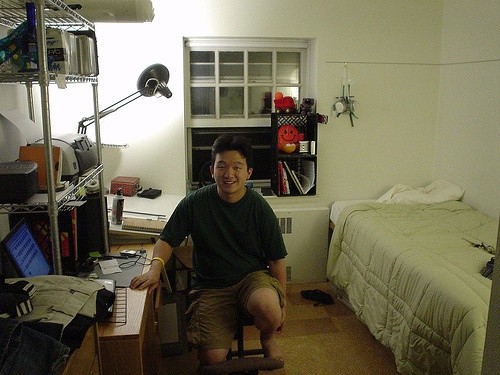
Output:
[111, 188, 125, 225]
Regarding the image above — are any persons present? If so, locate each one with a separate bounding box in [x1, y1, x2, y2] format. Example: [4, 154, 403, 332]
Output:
[129, 134, 287, 367]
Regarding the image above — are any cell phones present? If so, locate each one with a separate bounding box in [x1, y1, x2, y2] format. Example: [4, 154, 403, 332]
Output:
[120, 250, 136, 257]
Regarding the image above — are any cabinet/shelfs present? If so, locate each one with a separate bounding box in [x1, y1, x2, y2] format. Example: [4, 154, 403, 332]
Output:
[266, 198, 330, 285]
[61, 321, 101, 375]
[1, 1, 109, 275]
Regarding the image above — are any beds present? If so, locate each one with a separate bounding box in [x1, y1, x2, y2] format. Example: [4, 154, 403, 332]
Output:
[326, 200, 500, 375]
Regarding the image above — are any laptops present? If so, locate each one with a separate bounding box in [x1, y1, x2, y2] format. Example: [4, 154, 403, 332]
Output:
[2, 218, 115, 315]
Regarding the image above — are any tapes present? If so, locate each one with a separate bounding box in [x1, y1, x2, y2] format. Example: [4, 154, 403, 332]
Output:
[87, 180, 99, 191]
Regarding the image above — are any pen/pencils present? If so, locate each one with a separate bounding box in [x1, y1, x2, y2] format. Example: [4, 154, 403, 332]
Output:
[105, 255, 128, 259]
[103, 257, 113, 260]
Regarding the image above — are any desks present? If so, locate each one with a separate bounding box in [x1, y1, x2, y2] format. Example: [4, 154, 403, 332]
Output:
[92, 241, 161, 375]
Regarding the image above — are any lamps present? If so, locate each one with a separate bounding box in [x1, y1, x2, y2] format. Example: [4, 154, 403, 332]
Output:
[77, 64, 172, 134]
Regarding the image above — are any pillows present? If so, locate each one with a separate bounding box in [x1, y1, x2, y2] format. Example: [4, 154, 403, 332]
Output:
[377, 180, 464, 206]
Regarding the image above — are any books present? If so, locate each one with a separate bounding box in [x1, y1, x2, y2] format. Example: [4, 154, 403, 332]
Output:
[278, 160, 315, 195]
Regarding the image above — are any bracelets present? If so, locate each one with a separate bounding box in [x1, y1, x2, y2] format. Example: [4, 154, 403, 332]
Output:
[151, 257, 164, 269]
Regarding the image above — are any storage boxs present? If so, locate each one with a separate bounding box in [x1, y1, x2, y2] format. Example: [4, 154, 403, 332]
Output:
[272, 112, 317, 197]
[111, 176, 141, 197]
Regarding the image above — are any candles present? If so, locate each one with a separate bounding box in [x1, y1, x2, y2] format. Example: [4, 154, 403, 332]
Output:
[342, 62, 352, 97]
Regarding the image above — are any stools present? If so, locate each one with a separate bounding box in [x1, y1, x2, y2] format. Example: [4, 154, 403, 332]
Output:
[227, 323, 264, 361]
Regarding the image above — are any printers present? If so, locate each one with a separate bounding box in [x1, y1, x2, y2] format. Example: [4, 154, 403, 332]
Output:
[27, 130, 98, 180]
